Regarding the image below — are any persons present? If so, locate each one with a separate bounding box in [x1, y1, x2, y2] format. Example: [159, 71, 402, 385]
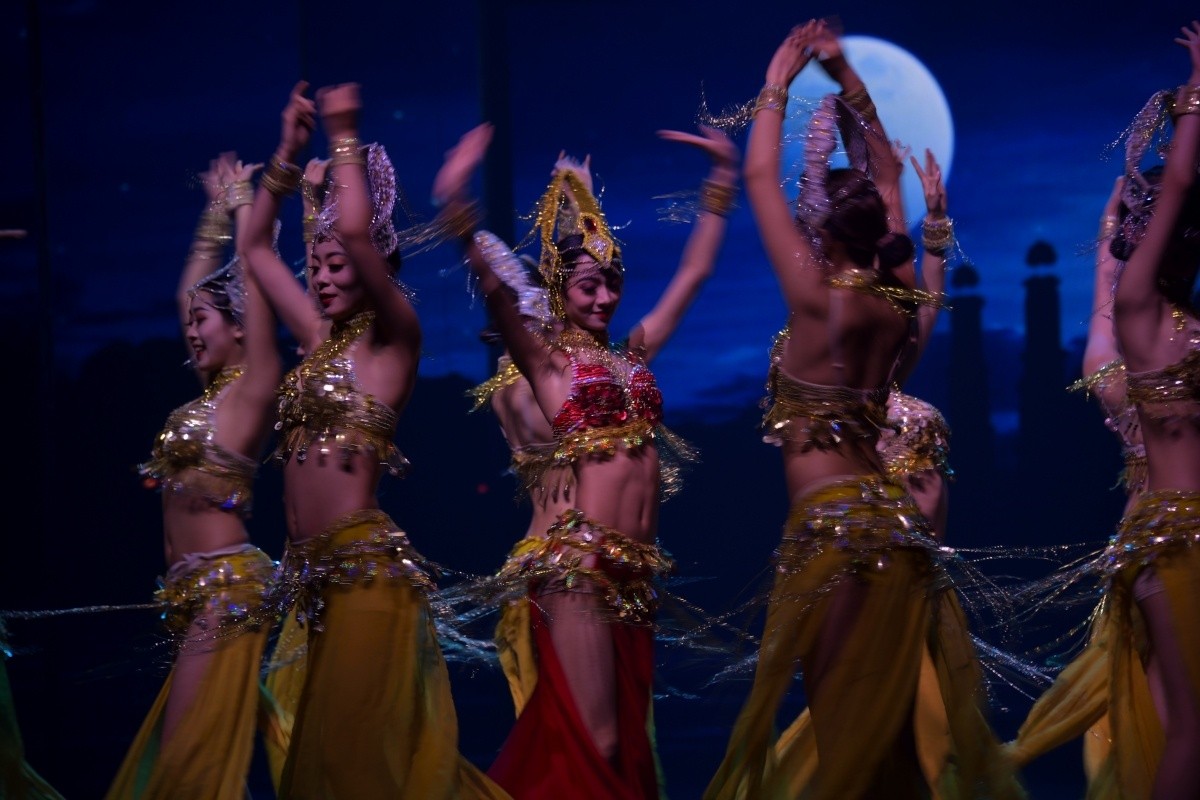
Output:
[996, 175, 1164, 800]
[107, 151, 280, 800]
[874, 153, 956, 541]
[698, 17, 1027, 800]
[1098, 20, 1200, 800]
[495, 347, 578, 720]
[430, 122, 740, 800]
[240, 82, 514, 800]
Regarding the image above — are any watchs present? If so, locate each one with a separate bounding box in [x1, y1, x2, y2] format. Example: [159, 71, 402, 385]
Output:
[752, 85, 788, 119]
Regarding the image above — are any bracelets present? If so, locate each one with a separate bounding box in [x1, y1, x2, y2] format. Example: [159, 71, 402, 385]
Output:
[262, 155, 303, 196]
[329, 137, 362, 167]
[1100, 216, 1121, 239]
[445, 202, 480, 237]
[1171, 84, 1200, 118]
[194, 212, 230, 244]
[838, 84, 876, 123]
[923, 215, 950, 249]
[225, 180, 255, 214]
[698, 179, 738, 218]
